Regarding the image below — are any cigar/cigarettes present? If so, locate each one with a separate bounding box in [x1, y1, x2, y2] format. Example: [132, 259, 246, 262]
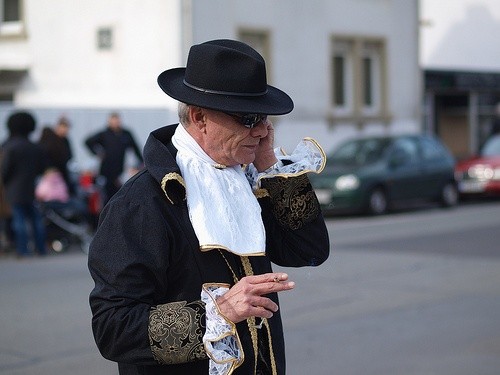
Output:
[274, 276, 280, 284]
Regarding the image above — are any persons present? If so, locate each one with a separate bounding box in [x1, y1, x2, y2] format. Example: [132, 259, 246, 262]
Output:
[83, 113, 145, 205]
[87, 39, 331, 375]
[0, 111, 78, 257]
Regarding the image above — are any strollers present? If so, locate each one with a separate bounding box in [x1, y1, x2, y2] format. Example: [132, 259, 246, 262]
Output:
[44, 200, 92, 254]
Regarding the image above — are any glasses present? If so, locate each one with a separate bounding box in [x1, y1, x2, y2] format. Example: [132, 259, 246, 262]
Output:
[225, 112, 267, 128]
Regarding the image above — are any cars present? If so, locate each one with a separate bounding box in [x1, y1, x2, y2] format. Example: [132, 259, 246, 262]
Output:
[454, 134, 499, 199]
[306, 134, 460, 216]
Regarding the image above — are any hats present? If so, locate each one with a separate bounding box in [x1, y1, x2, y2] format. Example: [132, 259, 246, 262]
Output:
[156, 39, 294, 115]
